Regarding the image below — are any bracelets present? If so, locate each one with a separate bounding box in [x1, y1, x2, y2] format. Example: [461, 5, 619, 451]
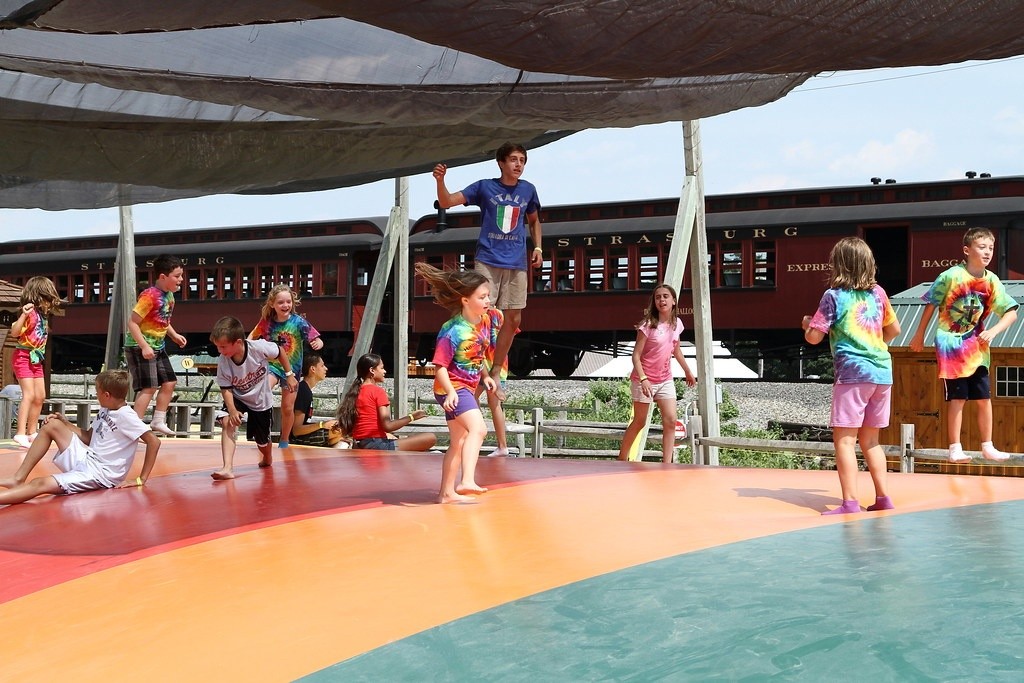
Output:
[408, 413, 414, 421]
[136, 476, 142, 486]
[319, 420, 324, 428]
[640, 375, 647, 383]
[285, 370, 293, 376]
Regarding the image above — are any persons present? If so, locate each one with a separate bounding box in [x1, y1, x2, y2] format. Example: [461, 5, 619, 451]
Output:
[11, 276, 70, 449]
[616, 284, 696, 463]
[433, 141, 543, 401]
[210, 316, 299, 480]
[909, 227, 1020, 463]
[414, 262, 497, 504]
[278, 355, 341, 447]
[474, 306, 521, 457]
[247, 284, 323, 448]
[0, 369, 161, 504]
[802, 237, 901, 515]
[124, 253, 187, 436]
[337, 353, 437, 451]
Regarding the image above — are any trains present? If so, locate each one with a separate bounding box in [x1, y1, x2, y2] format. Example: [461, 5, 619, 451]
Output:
[0, 172, 1024, 380]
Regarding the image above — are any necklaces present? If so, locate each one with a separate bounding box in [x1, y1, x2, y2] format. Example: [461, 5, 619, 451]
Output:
[534, 247, 543, 253]
